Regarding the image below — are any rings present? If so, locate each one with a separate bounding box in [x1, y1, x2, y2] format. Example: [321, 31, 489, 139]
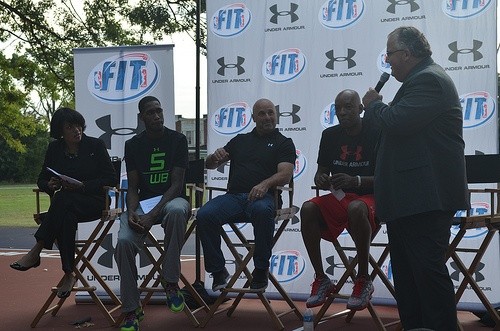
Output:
[257, 194, 259, 195]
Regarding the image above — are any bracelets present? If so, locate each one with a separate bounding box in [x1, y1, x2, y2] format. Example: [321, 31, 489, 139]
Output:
[355, 175, 362, 190]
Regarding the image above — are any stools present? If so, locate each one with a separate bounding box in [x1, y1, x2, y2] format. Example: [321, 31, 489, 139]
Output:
[111, 158, 211, 328]
[30, 156, 122, 329]
[310, 185, 401, 331]
[198, 170, 306, 331]
[445, 152, 500, 331]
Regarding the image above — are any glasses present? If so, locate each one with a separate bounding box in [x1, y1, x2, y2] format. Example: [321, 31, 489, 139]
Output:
[386, 48, 415, 58]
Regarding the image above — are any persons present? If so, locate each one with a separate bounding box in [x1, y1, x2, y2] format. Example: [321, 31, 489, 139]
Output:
[195, 98, 297, 292]
[362, 26, 472, 331]
[10, 107, 115, 298]
[300, 89, 382, 310]
[115, 96, 191, 331]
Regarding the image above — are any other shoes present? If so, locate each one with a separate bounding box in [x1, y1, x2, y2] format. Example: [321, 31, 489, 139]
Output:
[57, 275, 75, 298]
[10, 255, 41, 271]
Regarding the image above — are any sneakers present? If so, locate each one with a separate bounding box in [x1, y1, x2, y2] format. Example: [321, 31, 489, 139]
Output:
[305, 273, 336, 309]
[250, 268, 269, 292]
[347, 274, 374, 311]
[209, 267, 230, 291]
[120, 305, 144, 331]
[159, 276, 184, 313]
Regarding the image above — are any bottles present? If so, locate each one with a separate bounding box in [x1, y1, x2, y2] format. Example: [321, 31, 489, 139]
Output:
[304, 308, 313, 331]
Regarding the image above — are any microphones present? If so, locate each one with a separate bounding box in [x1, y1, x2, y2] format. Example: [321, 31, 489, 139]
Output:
[375, 72, 390, 92]
